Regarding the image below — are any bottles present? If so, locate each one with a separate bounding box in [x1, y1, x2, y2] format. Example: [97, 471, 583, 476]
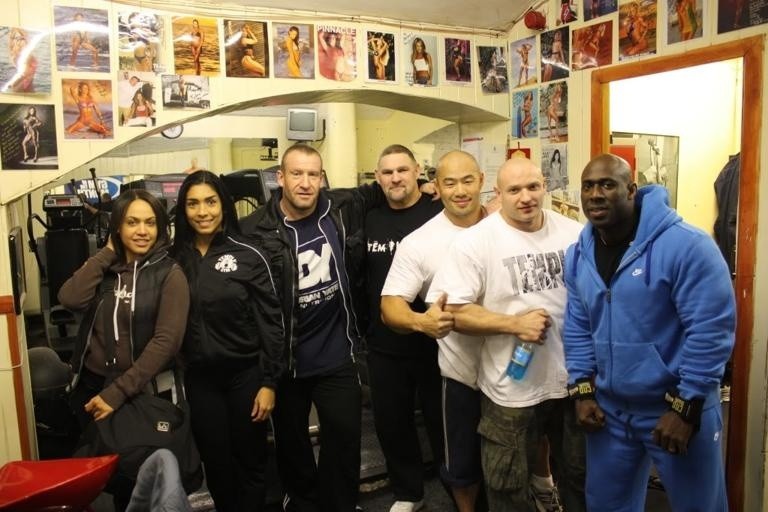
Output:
[503, 336, 537, 378]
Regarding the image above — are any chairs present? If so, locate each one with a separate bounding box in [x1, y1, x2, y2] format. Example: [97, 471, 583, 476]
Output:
[0, 455, 119, 512]
[124, 448, 194, 512]
[28, 346, 74, 428]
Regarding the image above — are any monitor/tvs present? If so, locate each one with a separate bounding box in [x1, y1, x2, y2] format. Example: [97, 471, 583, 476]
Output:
[286, 108, 318, 141]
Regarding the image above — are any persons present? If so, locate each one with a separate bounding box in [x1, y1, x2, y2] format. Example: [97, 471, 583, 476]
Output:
[59, 146, 740, 512]
[0, 1, 768, 164]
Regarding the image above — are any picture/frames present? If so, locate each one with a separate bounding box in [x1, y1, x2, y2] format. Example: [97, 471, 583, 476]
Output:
[8, 226, 28, 316]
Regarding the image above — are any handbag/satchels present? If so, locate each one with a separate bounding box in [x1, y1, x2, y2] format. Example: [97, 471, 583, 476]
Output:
[74, 394, 203, 498]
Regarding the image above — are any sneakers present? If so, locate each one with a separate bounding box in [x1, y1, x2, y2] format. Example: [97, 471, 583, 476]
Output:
[390, 500, 423, 512]
